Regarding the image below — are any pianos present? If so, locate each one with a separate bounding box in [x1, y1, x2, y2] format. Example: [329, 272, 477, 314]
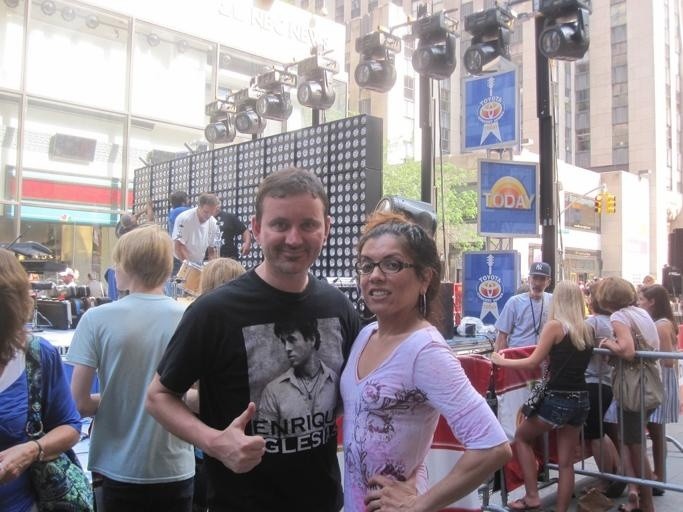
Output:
[19, 261, 66, 274]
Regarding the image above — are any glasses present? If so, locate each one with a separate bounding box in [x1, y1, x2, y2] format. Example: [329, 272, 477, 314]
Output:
[354, 258, 417, 276]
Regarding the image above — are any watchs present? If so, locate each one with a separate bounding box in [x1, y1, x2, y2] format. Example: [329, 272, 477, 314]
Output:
[32, 439, 45, 465]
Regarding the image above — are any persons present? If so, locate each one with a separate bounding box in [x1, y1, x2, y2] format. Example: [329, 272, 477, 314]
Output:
[489, 281, 592, 512]
[497, 260, 676, 497]
[162, 191, 251, 299]
[255, 315, 338, 443]
[597, 277, 660, 511]
[0, 246, 82, 512]
[67, 223, 200, 511]
[336, 210, 511, 512]
[143, 165, 363, 512]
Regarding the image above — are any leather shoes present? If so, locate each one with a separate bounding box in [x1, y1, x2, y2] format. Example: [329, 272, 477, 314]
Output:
[619, 503, 642, 512]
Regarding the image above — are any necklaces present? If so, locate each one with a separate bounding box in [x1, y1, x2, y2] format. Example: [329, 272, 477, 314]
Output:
[296, 373, 320, 401]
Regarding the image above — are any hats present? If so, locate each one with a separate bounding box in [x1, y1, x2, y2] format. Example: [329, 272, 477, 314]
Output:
[60, 268, 73, 276]
[529, 262, 551, 278]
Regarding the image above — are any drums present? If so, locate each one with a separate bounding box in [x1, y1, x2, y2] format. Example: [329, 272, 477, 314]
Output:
[174, 260, 202, 296]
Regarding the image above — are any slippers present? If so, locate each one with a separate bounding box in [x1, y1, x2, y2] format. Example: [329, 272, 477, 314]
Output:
[608, 477, 626, 499]
[508, 497, 540, 510]
[652, 488, 666, 496]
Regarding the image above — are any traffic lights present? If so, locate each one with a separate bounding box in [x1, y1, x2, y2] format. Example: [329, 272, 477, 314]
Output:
[607, 192, 616, 215]
[593, 193, 603, 215]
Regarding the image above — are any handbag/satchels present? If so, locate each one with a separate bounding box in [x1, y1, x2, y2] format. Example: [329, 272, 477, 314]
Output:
[611, 342, 662, 412]
[29, 447, 94, 511]
[521, 385, 545, 417]
[576, 488, 614, 512]
